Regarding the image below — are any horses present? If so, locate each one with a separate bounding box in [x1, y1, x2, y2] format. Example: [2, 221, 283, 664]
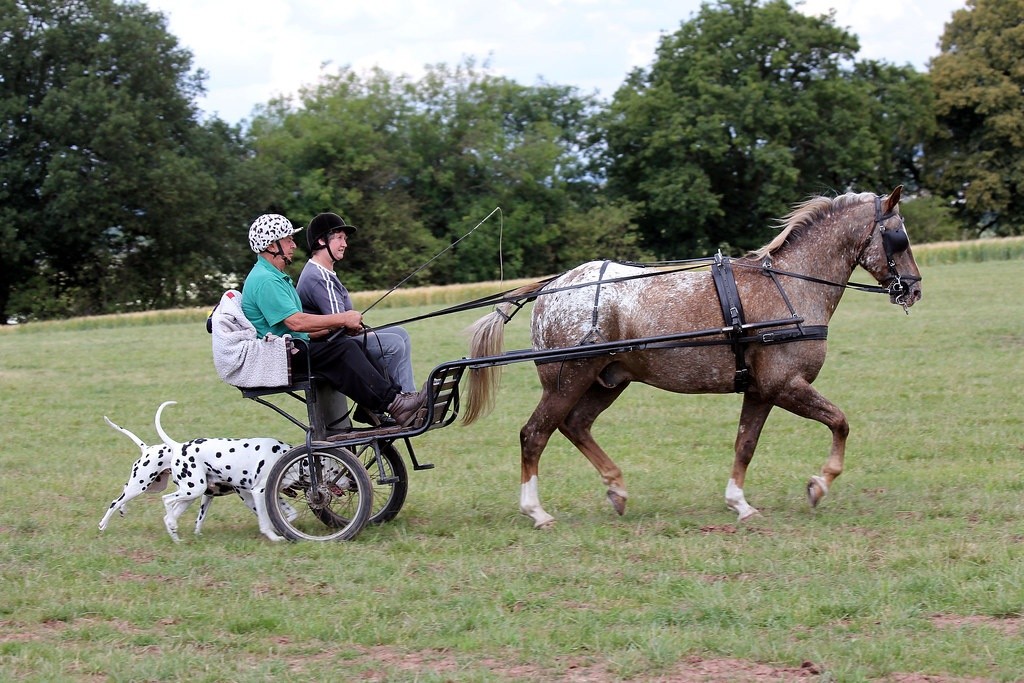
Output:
[453, 184, 923, 532]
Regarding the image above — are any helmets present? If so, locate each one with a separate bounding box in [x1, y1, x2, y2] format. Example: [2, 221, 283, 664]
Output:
[306, 212, 357, 252]
[248, 213, 304, 254]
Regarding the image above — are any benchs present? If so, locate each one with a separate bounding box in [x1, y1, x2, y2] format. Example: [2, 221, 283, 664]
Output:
[212, 290, 469, 446]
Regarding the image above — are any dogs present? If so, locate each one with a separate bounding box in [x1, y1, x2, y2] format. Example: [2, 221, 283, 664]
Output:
[96, 415, 302, 535]
[154, 400, 355, 544]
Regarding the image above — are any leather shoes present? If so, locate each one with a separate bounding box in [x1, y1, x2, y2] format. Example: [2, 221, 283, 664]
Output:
[388, 381, 429, 427]
[353, 405, 397, 427]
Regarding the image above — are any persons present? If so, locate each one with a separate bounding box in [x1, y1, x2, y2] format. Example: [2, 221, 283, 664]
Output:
[295, 213, 420, 425]
[241, 213, 428, 428]
